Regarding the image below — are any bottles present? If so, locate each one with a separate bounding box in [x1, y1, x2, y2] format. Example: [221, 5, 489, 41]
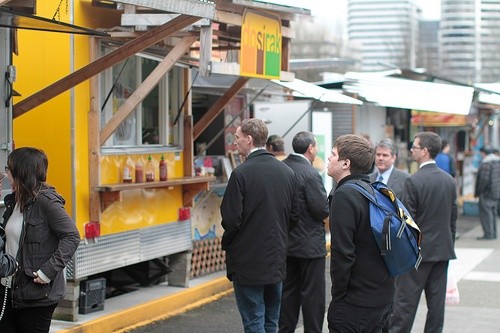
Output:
[145, 154, 155, 182]
[135, 159, 143, 183]
[122, 165, 132, 182]
[159, 154, 168, 181]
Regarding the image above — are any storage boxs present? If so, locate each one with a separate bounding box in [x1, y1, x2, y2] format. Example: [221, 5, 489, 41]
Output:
[79, 277, 106, 315]
[462, 199, 480, 217]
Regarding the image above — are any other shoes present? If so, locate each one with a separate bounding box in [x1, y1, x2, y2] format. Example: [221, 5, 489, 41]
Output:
[476, 235, 497, 240]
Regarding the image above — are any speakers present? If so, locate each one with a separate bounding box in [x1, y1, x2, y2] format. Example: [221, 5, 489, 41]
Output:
[79, 277, 106, 314]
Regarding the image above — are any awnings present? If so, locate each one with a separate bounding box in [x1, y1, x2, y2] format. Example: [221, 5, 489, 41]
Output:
[266, 78, 365, 106]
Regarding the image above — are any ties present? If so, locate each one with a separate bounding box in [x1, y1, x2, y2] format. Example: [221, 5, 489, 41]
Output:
[379, 176, 384, 182]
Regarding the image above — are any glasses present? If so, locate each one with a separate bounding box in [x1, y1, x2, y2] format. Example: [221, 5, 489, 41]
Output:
[412, 144, 422, 150]
[5, 167, 11, 171]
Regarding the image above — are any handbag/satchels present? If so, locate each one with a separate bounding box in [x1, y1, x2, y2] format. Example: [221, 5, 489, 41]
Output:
[444, 259, 460, 304]
[343, 180, 423, 277]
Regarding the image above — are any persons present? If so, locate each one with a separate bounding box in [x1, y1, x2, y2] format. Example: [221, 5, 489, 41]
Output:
[0, 146, 81, 333]
[278, 131, 329, 333]
[219, 118, 301, 333]
[369, 139, 411, 203]
[266, 135, 287, 163]
[389, 132, 459, 333]
[473, 145, 500, 241]
[327, 134, 395, 333]
